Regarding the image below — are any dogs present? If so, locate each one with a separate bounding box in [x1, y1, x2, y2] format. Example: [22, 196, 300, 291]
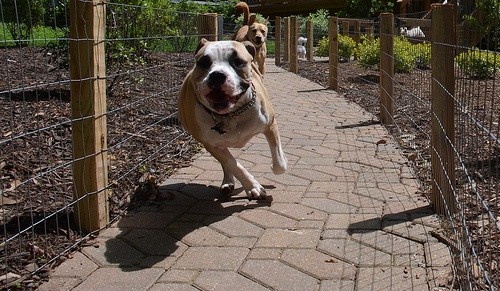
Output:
[178, 37, 288, 202]
[400, 26, 425, 37]
[297, 37, 307, 60]
[235, 2, 269, 77]
